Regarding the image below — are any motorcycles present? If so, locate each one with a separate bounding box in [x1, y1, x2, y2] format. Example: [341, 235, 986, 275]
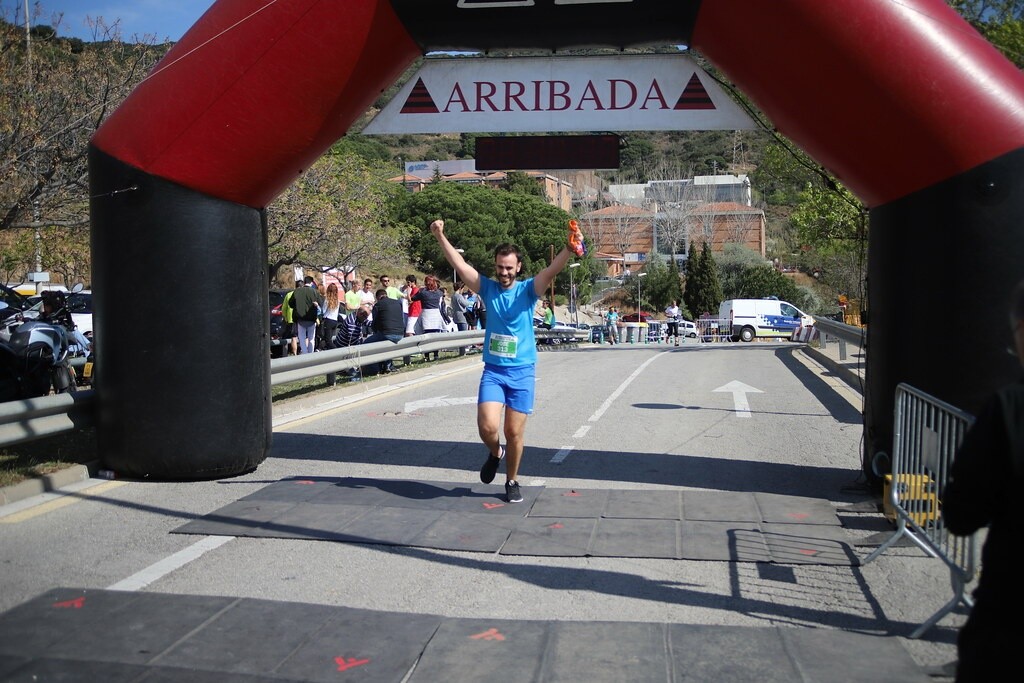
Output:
[0, 282, 84, 404]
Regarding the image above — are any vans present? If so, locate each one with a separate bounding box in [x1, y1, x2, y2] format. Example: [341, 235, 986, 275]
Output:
[718, 299, 816, 342]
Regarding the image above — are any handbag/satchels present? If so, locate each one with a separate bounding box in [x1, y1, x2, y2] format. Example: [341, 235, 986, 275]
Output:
[463, 308, 477, 325]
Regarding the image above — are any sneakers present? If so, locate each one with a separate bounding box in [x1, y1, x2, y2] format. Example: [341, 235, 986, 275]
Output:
[480, 445, 505, 483]
[505, 480, 524, 502]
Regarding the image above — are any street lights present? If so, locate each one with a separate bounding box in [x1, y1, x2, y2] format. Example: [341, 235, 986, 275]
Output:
[637, 273, 647, 322]
[569, 263, 580, 323]
[453, 249, 464, 283]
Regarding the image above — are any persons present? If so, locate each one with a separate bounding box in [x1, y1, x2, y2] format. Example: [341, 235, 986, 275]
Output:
[430, 218, 586, 503]
[703, 312, 711, 328]
[665, 300, 680, 346]
[536, 300, 556, 346]
[604, 306, 619, 345]
[941, 278, 1024, 683]
[282, 275, 487, 382]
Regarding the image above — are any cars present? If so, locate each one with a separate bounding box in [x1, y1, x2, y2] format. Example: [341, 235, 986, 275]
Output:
[0, 281, 33, 322]
[0, 290, 93, 349]
[613, 274, 632, 283]
[661, 320, 699, 338]
[443, 305, 459, 332]
[621, 312, 654, 323]
[532, 317, 609, 346]
[775, 264, 796, 272]
[268, 289, 292, 339]
[595, 276, 625, 285]
[648, 322, 665, 341]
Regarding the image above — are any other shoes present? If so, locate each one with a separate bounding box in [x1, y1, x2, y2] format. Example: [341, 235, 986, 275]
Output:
[675, 343, 679, 346]
[666, 336, 670, 343]
[349, 351, 466, 380]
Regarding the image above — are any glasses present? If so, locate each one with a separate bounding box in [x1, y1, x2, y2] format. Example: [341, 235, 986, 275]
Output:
[383, 280, 389, 282]
